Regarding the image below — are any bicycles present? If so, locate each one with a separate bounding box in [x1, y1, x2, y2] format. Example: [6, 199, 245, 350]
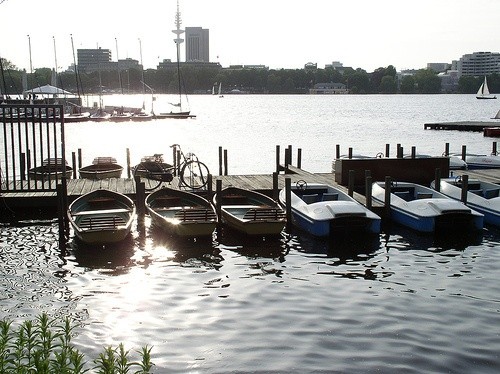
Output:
[133, 143, 210, 189]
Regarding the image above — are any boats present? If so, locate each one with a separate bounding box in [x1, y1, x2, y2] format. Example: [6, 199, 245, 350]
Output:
[212, 184, 287, 236]
[143, 184, 219, 237]
[78, 156, 123, 180]
[278, 179, 381, 242]
[371, 181, 485, 234]
[131, 160, 173, 177]
[429, 175, 500, 227]
[66, 186, 137, 245]
[28, 156, 73, 179]
[331, 151, 500, 170]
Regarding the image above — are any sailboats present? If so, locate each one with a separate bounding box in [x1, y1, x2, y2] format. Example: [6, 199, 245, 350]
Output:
[0, 32, 197, 122]
[476, 75, 498, 99]
[212, 81, 224, 98]
